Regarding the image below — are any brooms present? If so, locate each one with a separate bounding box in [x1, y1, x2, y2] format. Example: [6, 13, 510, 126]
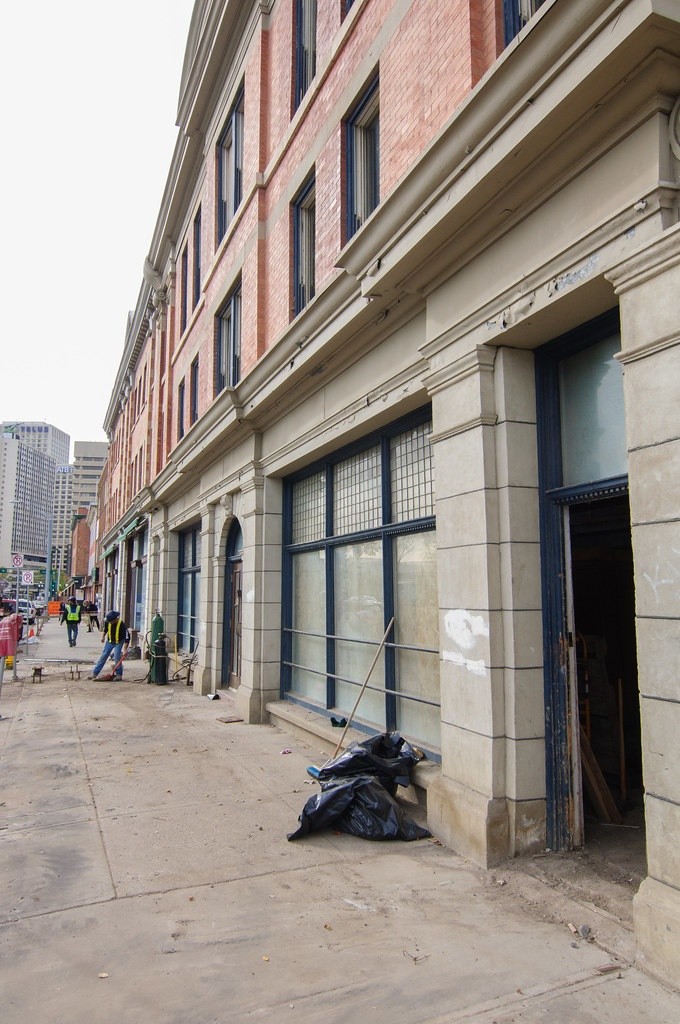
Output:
[305, 616, 396, 781]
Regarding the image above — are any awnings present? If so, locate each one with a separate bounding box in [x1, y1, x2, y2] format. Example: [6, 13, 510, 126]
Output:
[117, 518, 140, 546]
[98, 542, 115, 562]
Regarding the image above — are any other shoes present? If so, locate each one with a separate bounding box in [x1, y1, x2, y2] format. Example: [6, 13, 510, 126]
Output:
[68, 640, 73, 646]
[72, 640, 76, 645]
[87, 675, 95, 680]
[113, 675, 122, 681]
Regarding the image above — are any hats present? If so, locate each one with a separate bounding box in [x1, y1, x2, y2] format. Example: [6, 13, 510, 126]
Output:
[68, 596, 76, 601]
[105, 610, 120, 621]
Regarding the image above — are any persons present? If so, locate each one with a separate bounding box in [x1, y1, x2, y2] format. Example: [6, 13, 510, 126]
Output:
[85, 611, 130, 681]
[59, 595, 99, 648]
